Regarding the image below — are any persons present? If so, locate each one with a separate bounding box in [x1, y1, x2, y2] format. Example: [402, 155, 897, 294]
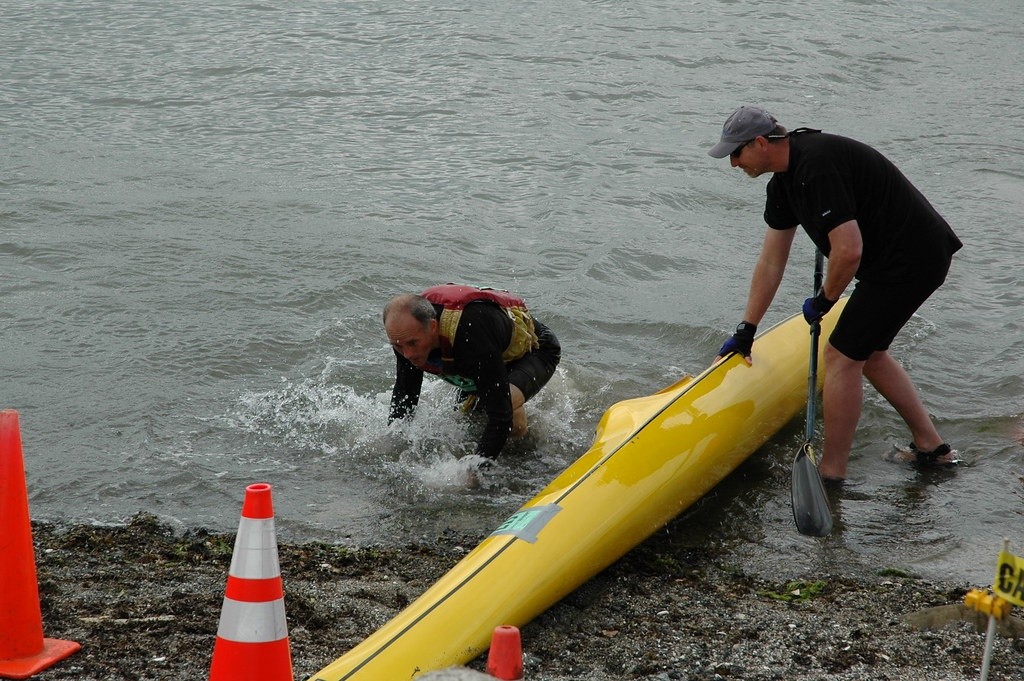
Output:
[711, 106, 963, 493]
[384, 283, 561, 467]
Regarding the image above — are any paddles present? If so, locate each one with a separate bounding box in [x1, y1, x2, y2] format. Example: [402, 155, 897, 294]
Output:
[788, 248, 836, 539]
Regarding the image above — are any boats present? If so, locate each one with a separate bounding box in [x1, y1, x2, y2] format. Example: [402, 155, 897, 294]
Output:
[302, 284, 851, 681]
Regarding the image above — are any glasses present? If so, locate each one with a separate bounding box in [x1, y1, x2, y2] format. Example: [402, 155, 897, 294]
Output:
[729, 139, 755, 158]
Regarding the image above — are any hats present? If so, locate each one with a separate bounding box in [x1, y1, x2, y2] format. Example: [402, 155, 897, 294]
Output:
[707, 105, 776, 159]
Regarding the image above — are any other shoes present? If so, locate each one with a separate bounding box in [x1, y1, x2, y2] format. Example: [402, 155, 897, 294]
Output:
[821, 477, 845, 493]
[881, 442, 960, 468]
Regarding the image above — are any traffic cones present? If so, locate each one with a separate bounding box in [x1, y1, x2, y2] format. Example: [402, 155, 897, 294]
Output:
[0, 405, 83, 678]
[208, 482, 296, 681]
[486, 623, 527, 678]
